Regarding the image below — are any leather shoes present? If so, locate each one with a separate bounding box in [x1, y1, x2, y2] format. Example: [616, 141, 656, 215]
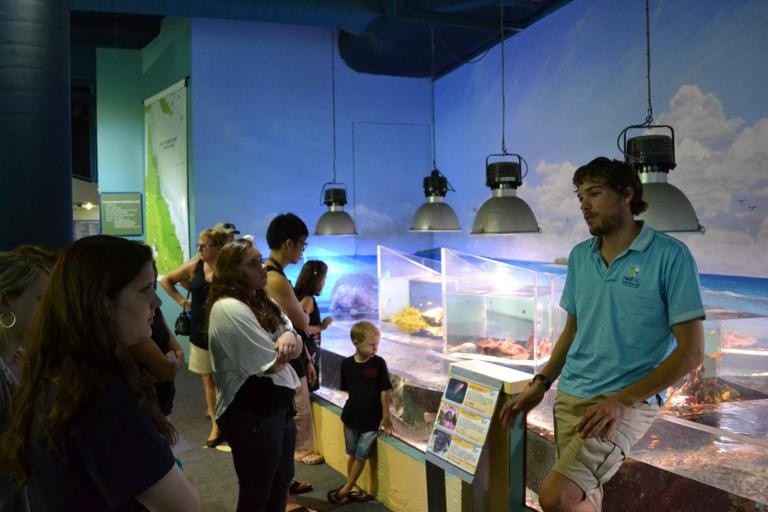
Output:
[207, 432, 221, 449]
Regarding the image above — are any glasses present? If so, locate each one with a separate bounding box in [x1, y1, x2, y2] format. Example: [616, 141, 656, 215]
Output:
[245, 256, 265, 268]
[196, 243, 218, 251]
[292, 238, 310, 248]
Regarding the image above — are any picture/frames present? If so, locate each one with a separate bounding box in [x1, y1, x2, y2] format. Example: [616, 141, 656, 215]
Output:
[99, 192, 143, 236]
[141, 74, 191, 281]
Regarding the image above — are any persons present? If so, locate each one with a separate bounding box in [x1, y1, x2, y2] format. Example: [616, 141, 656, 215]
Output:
[0, 243, 60, 420]
[265, 212, 314, 512]
[153, 260, 184, 421]
[213, 221, 240, 238]
[294, 261, 332, 391]
[501, 155, 707, 512]
[208, 238, 303, 512]
[0, 236, 203, 512]
[330, 319, 394, 503]
[160, 228, 228, 447]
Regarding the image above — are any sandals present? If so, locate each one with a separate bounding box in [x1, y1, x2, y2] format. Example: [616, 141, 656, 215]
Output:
[294, 449, 324, 465]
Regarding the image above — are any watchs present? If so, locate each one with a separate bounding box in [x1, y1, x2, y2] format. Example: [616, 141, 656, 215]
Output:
[530, 374, 552, 391]
[284, 328, 298, 339]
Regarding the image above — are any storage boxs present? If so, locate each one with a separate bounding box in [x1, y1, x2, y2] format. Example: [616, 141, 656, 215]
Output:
[373, 244, 443, 337]
[442, 243, 567, 369]
[658, 305, 768, 440]
[302, 243, 376, 331]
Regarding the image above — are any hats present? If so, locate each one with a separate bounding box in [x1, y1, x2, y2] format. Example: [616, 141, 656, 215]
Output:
[215, 221, 240, 236]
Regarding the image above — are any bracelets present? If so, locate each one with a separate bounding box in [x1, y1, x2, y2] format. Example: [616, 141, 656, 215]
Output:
[179, 299, 187, 305]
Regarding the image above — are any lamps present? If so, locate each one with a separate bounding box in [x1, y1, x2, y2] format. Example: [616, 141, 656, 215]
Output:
[616, 0, 707, 237]
[407, 26, 463, 233]
[73, 200, 98, 211]
[314, 23, 357, 236]
[469, 0, 542, 236]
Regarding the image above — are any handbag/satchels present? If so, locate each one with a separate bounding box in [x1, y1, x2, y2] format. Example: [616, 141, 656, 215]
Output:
[175, 284, 193, 336]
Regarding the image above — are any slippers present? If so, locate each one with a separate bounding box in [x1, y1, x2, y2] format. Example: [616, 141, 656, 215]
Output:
[289, 479, 314, 493]
[328, 486, 348, 502]
[288, 503, 319, 512]
[348, 484, 370, 501]
